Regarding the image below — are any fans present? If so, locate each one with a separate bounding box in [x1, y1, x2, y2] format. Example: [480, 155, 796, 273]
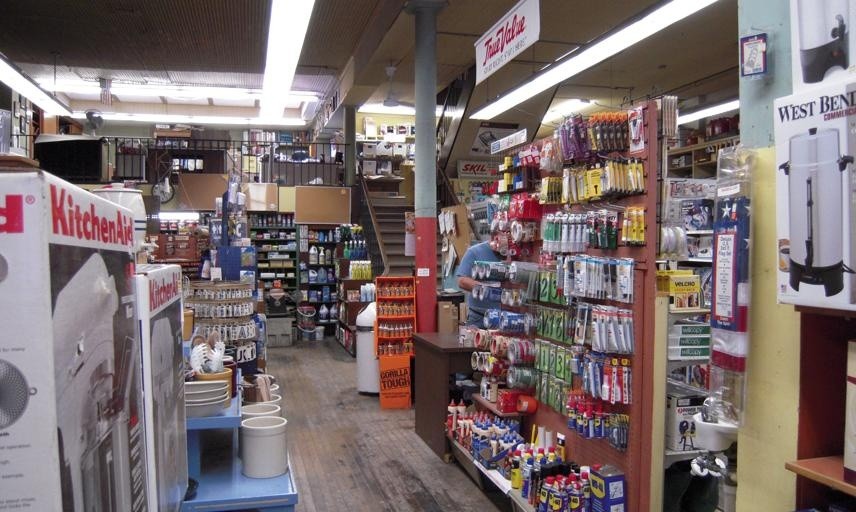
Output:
[81, 108, 104, 136]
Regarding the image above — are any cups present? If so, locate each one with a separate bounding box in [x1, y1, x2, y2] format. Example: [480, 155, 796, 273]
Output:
[239, 374, 288, 482]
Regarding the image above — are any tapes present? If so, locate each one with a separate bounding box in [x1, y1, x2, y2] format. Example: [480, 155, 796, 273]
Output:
[470, 211, 539, 390]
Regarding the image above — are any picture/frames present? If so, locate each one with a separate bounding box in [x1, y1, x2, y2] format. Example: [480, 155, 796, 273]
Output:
[13, 94, 31, 135]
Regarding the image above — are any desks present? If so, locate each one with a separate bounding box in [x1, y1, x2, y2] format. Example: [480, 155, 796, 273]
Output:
[259, 155, 340, 187]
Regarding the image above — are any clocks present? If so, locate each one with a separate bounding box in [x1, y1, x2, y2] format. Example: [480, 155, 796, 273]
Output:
[151, 181, 176, 205]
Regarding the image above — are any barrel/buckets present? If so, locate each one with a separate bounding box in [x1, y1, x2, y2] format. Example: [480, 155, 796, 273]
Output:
[318, 303, 329, 323]
[184, 355, 289, 479]
[308, 244, 320, 265]
[317, 267, 328, 286]
[297, 306, 316, 327]
[296, 326, 315, 343]
[330, 304, 338, 324]
[314, 325, 326, 341]
[308, 268, 317, 284]
[327, 267, 335, 283]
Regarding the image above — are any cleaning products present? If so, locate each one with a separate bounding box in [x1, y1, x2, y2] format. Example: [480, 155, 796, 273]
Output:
[318, 246, 326, 264]
[339, 222, 376, 303]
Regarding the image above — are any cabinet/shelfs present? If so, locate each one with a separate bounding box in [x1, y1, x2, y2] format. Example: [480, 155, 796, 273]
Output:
[409, 327, 479, 468]
[663, 183, 717, 475]
[239, 215, 299, 316]
[299, 221, 349, 335]
[333, 237, 371, 354]
[373, 264, 418, 361]
[780, 299, 856, 511]
[662, 133, 742, 182]
[175, 367, 299, 512]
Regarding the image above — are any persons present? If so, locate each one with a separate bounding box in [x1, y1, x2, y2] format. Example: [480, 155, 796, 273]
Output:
[458, 236, 511, 331]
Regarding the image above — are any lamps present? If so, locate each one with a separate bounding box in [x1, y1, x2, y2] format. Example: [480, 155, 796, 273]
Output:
[0, 48, 73, 117]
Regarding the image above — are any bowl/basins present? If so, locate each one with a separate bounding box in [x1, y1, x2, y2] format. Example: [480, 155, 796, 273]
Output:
[182, 379, 228, 420]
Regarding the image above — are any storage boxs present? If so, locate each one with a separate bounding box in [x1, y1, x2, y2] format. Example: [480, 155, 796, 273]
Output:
[0, 169, 153, 512]
[665, 392, 705, 452]
[787, 0, 855, 94]
[130, 259, 189, 512]
[355, 124, 414, 183]
[771, 75, 856, 315]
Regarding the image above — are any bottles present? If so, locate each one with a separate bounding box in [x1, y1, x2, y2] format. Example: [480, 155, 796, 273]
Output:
[308, 228, 337, 264]
[377, 322, 412, 338]
[777, 125, 854, 296]
[377, 340, 412, 356]
[377, 299, 415, 317]
[445, 395, 609, 512]
[249, 213, 295, 228]
[377, 279, 414, 297]
[343, 238, 371, 280]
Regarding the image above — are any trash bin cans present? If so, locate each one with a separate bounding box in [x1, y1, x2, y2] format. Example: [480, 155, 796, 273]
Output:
[356, 302, 380, 396]
